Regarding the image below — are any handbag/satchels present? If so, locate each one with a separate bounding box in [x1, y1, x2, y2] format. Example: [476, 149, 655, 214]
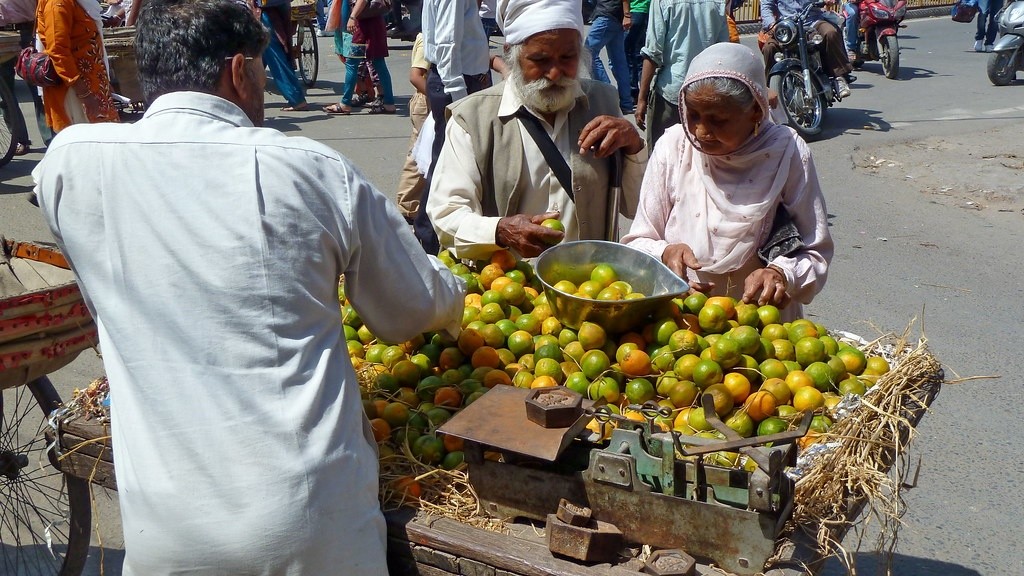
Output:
[349, 0, 392, 17]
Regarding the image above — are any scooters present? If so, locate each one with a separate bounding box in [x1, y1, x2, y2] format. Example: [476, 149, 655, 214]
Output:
[986, 0, 1024, 87]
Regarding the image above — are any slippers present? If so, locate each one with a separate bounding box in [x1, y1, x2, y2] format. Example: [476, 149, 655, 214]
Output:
[323, 103, 352, 115]
[280, 104, 311, 112]
[368, 106, 396, 114]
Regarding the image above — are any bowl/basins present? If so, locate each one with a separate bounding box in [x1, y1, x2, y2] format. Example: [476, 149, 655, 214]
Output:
[536, 240, 689, 334]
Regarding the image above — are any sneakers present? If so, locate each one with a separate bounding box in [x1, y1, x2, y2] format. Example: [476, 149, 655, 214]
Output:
[973, 39, 983, 52]
[835, 76, 851, 97]
[985, 45, 995, 53]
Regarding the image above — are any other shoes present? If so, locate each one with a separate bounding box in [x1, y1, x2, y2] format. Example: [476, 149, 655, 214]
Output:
[849, 51, 861, 63]
[15, 141, 31, 155]
[349, 92, 375, 106]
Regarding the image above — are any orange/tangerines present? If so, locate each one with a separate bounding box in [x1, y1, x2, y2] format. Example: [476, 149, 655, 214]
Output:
[338, 217, 891, 501]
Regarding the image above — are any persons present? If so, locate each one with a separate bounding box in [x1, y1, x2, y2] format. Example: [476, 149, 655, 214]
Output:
[974, 0, 1006, 53]
[618, 41, 834, 325]
[582, 0, 861, 163]
[0, 0, 510, 260]
[426, 0, 650, 272]
[31, 1, 466, 576]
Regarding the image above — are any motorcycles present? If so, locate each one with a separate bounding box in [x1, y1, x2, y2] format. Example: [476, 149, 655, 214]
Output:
[766, 0, 856, 140]
[838, 0, 909, 80]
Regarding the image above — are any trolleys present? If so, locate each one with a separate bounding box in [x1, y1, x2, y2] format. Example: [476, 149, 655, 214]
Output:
[289, 16, 319, 88]
[0, 371, 92, 576]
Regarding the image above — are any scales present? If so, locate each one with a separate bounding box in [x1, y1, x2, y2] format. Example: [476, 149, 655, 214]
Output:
[434, 384, 815, 576]
